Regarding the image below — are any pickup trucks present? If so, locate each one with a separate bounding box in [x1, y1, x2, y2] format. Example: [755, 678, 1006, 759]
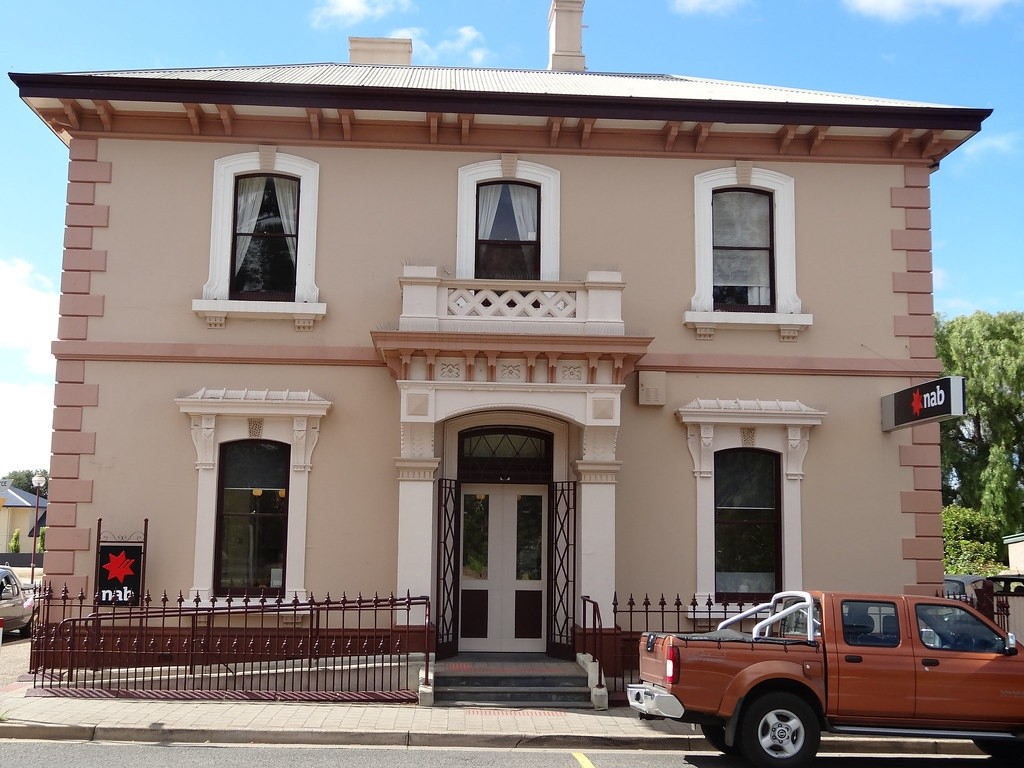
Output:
[0, 565, 41, 640]
[625, 589, 1024, 768]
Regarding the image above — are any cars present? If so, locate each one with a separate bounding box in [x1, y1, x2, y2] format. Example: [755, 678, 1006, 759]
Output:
[945, 574, 1006, 596]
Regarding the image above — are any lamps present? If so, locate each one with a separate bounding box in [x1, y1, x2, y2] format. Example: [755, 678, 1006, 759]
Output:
[278, 490, 286, 498]
[252, 489, 262, 496]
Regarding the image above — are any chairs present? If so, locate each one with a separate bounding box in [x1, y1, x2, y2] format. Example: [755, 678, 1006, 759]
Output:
[881, 616, 898, 643]
[849, 613, 882, 642]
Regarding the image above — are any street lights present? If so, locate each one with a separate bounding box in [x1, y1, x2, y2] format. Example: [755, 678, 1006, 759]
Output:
[31, 473, 46, 584]
